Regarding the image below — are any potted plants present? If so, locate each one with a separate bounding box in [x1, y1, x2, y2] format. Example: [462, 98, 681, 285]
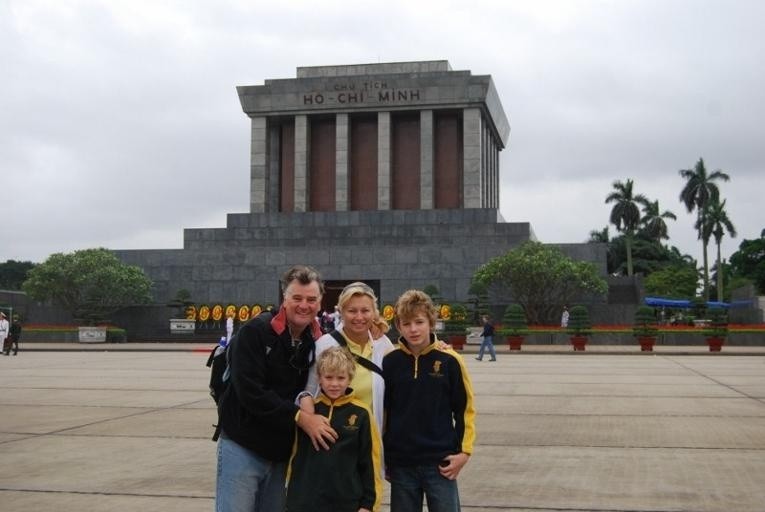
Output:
[445, 303, 468, 350]
[166, 289, 197, 335]
[567, 305, 592, 351]
[704, 308, 730, 352]
[503, 304, 529, 350]
[633, 308, 658, 351]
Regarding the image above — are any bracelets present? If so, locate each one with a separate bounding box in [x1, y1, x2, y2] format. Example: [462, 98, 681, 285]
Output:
[297, 392, 312, 399]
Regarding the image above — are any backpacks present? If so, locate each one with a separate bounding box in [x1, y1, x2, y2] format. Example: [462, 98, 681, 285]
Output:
[208, 310, 271, 404]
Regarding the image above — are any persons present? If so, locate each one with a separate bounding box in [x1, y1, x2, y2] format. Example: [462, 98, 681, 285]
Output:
[474, 315, 497, 362]
[293, 282, 454, 511]
[3, 314, 21, 355]
[0, 311, 10, 354]
[210, 263, 386, 512]
[280, 344, 384, 512]
[560, 305, 569, 328]
[385, 287, 476, 512]
